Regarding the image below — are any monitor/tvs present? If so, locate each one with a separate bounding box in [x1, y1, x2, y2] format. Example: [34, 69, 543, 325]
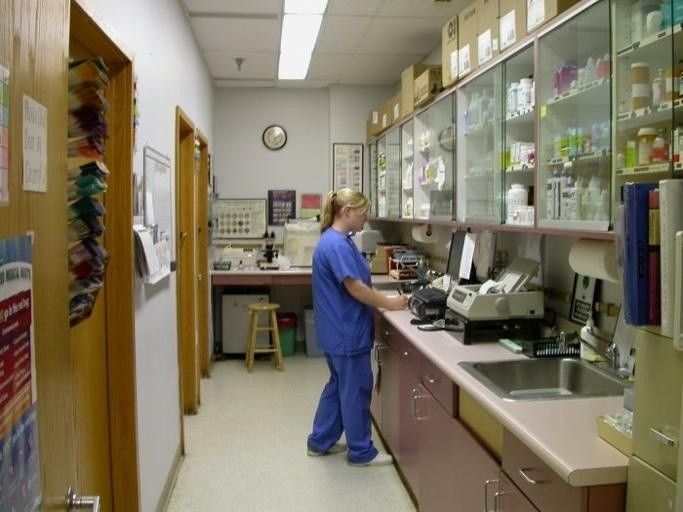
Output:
[447, 232, 466, 277]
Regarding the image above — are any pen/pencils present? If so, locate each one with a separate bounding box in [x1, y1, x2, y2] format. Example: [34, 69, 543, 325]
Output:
[396, 288, 402, 295]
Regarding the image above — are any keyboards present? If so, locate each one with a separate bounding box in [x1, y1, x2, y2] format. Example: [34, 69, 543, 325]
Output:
[413, 288, 447, 305]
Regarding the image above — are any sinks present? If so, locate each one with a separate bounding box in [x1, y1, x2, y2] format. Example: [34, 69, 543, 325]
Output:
[456, 356, 634, 401]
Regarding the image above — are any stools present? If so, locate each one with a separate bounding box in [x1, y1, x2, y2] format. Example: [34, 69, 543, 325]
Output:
[245, 302, 284, 372]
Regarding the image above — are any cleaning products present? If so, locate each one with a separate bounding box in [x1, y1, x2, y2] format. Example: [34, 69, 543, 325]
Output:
[579, 311, 599, 362]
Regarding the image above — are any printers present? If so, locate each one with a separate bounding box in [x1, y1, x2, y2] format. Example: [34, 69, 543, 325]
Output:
[444, 256, 545, 345]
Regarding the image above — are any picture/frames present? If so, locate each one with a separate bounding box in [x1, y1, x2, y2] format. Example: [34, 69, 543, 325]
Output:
[569, 272, 603, 326]
[210, 198, 269, 239]
[332, 143, 363, 201]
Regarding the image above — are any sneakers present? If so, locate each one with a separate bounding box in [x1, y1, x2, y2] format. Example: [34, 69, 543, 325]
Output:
[347, 452, 393, 467]
[307, 441, 346, 456]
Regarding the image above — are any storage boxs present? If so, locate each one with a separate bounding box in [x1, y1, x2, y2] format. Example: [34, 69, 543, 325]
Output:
[366, 0, 581, 143]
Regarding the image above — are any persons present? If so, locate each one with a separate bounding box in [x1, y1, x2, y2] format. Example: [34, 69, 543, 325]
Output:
[306, 187, 409, 467]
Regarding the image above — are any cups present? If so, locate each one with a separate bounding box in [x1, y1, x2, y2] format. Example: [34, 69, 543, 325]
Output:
[637, 125, 655, 166]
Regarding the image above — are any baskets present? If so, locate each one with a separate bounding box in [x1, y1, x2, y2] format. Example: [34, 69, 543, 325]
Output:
[522, 336, 580, 358]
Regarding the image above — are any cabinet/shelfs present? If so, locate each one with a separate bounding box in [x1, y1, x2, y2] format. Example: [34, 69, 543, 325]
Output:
[625, 327, 683, 512]
[366, 0, 683, 241]
[370, 313, 629, 511]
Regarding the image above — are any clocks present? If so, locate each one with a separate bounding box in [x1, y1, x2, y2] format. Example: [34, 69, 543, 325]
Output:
[263, 125, 288, 150]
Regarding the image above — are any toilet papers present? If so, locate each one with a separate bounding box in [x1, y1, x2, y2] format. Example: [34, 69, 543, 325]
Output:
[567, 237, 621, 285]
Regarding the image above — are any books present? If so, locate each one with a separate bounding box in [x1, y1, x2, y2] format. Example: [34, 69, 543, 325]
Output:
[67, 54, 110, 326]
[614, 177, 682, 353]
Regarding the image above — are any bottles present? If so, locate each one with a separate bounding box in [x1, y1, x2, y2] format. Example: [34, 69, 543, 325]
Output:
[629, 62, 650, 109]
[467, 77, 535, 127]
[506, 183, 530, 222]
[545, 54, 610, 158]
[652, 68, 667, 104]
[651, 127, 669, 163]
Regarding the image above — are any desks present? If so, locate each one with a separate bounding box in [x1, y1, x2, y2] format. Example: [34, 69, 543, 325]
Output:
[210, 269, 314, 353]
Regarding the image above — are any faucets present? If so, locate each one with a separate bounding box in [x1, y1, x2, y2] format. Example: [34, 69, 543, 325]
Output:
[557, 327, 621, 370]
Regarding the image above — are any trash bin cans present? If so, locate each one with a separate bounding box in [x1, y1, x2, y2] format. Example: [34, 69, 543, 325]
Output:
[276, 312, 298, 357]
[304, 305, 326, 358]
